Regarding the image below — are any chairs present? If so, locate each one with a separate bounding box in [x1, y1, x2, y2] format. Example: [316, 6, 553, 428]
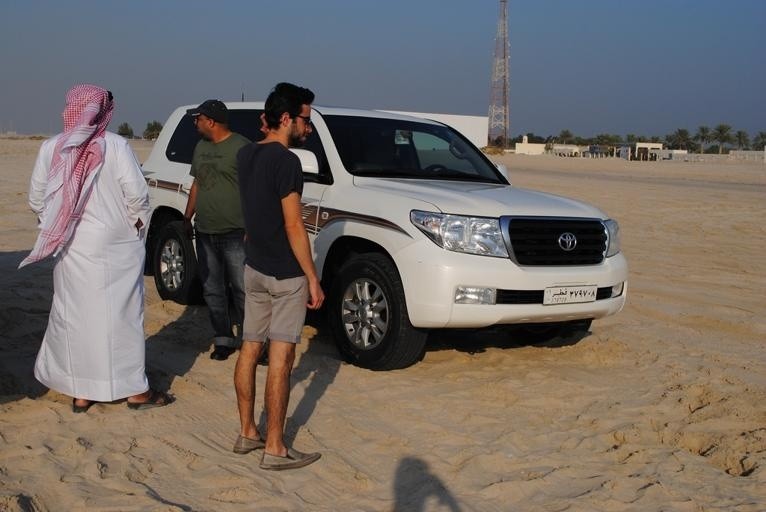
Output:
[351, 132, 415, 177]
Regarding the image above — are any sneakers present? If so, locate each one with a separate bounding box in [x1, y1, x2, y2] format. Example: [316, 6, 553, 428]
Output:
[259, 448, 321, 471]
[235, 435, 267, 454]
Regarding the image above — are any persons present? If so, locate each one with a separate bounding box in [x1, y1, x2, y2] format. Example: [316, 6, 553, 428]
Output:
[233, 82, 325, 470]
[181, 98, 273, 365]
[28, 83, 175, 413]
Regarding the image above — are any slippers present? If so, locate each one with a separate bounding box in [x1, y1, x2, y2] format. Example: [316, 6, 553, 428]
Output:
[71, 397, 90, 412]
[126, 393, 172, 409]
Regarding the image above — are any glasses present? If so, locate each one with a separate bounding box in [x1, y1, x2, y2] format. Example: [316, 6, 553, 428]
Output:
[298, 114, 311, 123]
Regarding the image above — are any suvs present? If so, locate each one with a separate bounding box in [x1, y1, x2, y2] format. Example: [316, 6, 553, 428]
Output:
[136, 101, 634, 377]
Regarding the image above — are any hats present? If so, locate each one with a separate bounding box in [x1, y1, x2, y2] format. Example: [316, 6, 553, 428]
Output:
[186, 99, 229, 123]
